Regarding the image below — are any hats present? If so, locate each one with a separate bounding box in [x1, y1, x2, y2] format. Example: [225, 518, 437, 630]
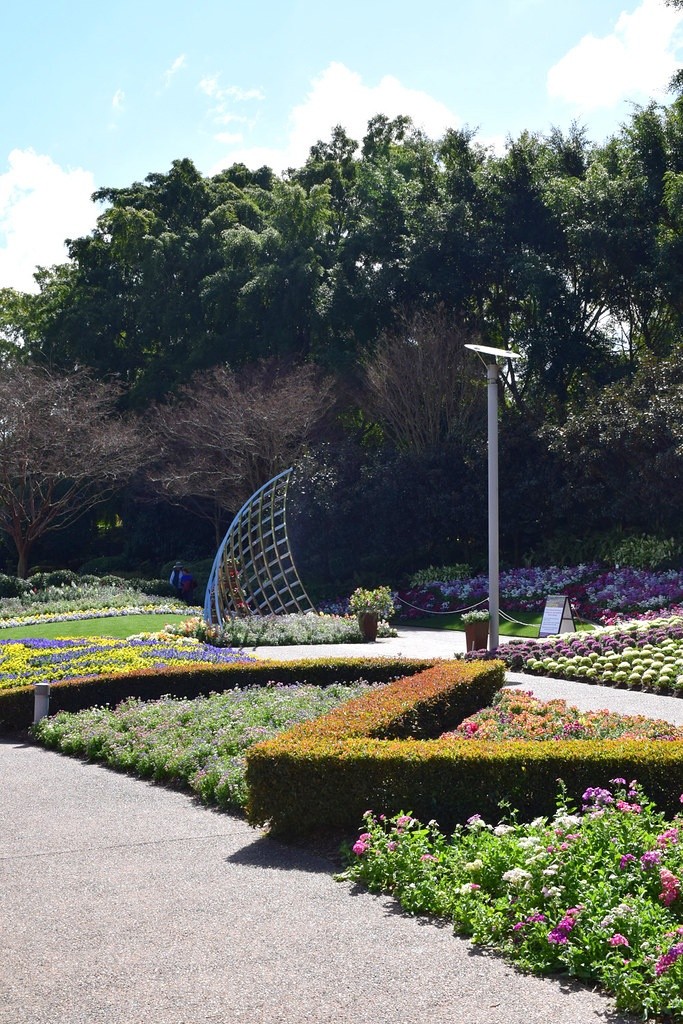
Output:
[173, 562, 183, 568]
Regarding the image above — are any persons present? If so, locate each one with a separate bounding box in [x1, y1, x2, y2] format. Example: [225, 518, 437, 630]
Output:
[170, 562, 186, 597]
[181, 568, 194, 606]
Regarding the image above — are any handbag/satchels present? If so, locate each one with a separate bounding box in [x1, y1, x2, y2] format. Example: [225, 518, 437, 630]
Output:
[192, 579, 198, 588]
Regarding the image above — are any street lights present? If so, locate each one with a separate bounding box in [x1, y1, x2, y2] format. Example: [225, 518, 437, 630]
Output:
[462, 341, 523, 651]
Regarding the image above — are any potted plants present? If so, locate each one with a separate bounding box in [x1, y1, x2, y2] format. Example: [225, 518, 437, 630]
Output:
[459, 609, 490, 653]
[348, 585, 395, 644]
[210, 558, 251, 624]
